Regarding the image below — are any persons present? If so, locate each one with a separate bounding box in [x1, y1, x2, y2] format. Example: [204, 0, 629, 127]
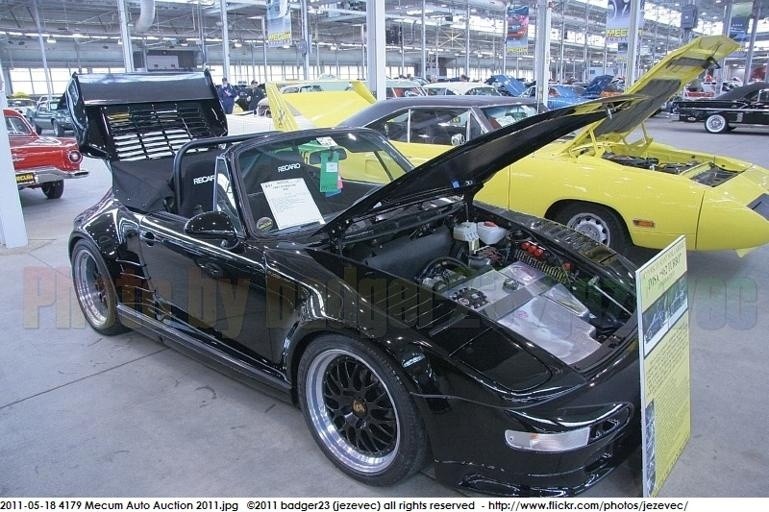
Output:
[430, 75, 438, 84]
[568, 76, 577, 84]
[219, 78, 236, 114]
[245, 80, 265, 114]
[459, 75, 467, 82]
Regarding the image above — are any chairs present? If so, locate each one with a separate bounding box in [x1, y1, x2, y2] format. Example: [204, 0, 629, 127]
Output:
[181, 151, 313, 229]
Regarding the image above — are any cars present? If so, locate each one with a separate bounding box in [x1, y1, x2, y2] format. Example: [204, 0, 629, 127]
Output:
[3, 95, 89, 198]
[68, 69, 693, 497]
